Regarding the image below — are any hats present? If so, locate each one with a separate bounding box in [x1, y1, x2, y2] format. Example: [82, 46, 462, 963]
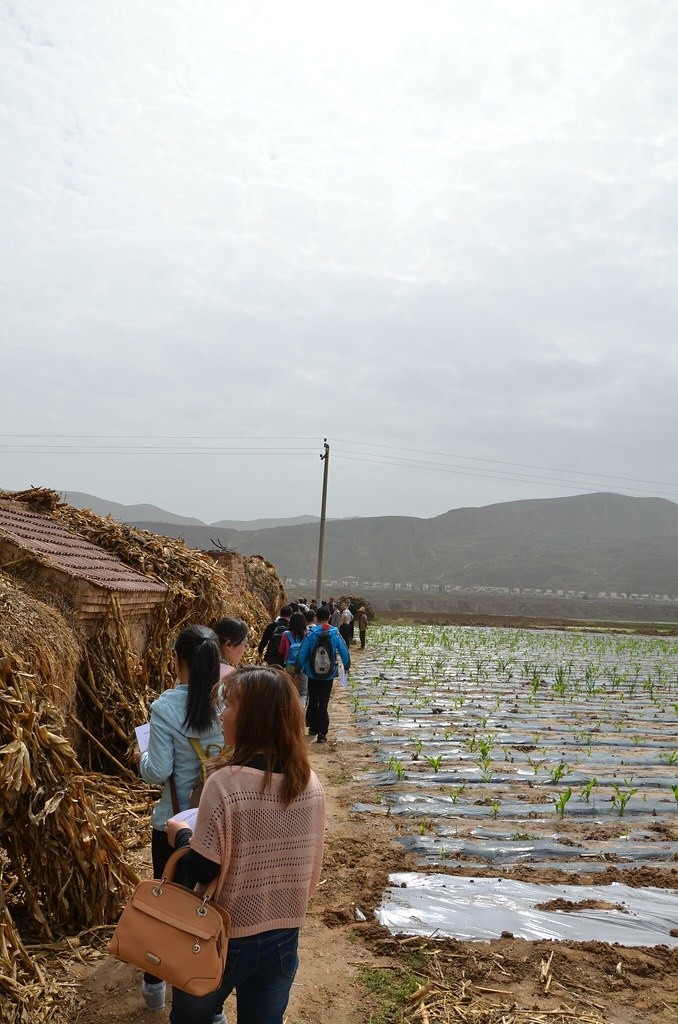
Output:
[359, 607, 366, 612]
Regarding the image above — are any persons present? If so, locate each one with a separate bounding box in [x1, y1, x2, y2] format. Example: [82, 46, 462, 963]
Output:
[166, 668, 324, 1024]
[135, 598, 371, 1024]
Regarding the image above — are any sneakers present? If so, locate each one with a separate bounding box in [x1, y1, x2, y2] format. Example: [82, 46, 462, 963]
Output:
[213, 1012, 228, 1024]
[142, 979, 166, 1009]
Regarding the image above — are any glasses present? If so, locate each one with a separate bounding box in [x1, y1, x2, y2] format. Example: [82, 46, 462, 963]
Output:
[237, 644, 250, 650]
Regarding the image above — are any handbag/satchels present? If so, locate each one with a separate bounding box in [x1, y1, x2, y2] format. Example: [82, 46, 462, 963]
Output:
[107, 846, 231, 997]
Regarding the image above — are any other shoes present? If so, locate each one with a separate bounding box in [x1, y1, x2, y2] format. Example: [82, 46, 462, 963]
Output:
[317, 733, 327, 743]
[308, 727, 318, 735]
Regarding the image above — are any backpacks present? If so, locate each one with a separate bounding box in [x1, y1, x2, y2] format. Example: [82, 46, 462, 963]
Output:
[309, 636, 337, 679]
[285, 631, 305, 675]
[170, 738, 235, 815]
[264, 620, 289, 667]
